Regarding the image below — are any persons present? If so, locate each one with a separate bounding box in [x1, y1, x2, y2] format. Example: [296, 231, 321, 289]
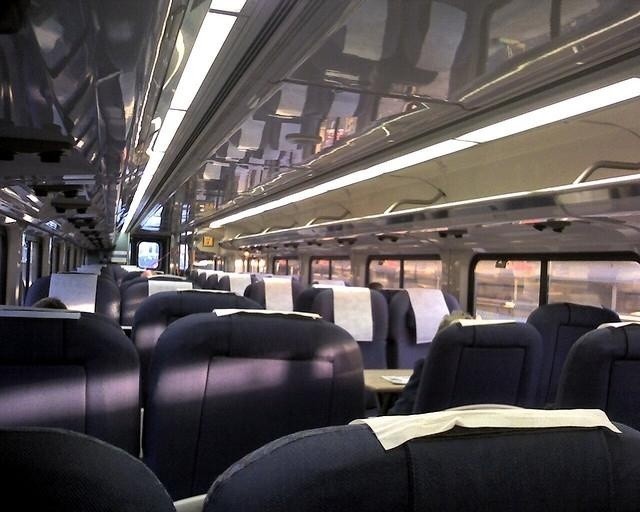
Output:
[32, 298, 68, 309]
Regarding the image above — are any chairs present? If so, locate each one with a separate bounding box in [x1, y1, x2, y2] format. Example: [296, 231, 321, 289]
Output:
[0, 263, 639, 512]
